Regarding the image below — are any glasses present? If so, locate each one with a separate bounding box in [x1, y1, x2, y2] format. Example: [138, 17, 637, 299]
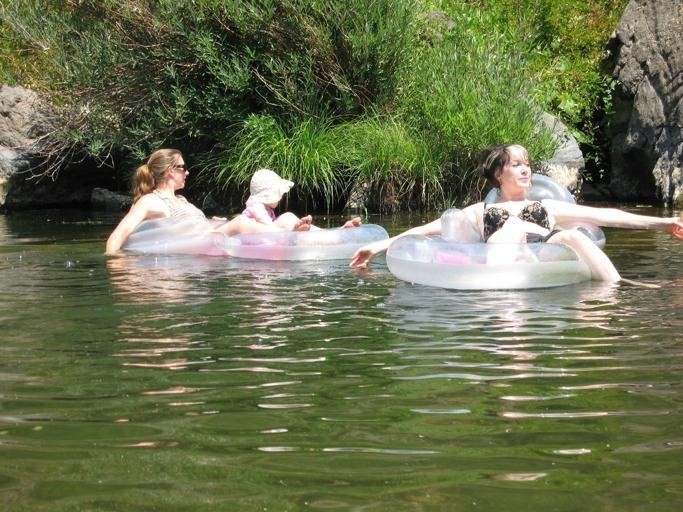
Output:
[173, 164, 187, 172]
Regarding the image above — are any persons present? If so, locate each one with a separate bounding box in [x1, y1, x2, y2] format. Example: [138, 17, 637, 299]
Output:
[241, 166, 361, 236]
[102, 145, 314, 263]
[349, 143, 681, 286]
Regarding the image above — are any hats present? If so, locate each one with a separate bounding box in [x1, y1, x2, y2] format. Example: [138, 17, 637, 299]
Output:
[245, 168, 295, 205]
